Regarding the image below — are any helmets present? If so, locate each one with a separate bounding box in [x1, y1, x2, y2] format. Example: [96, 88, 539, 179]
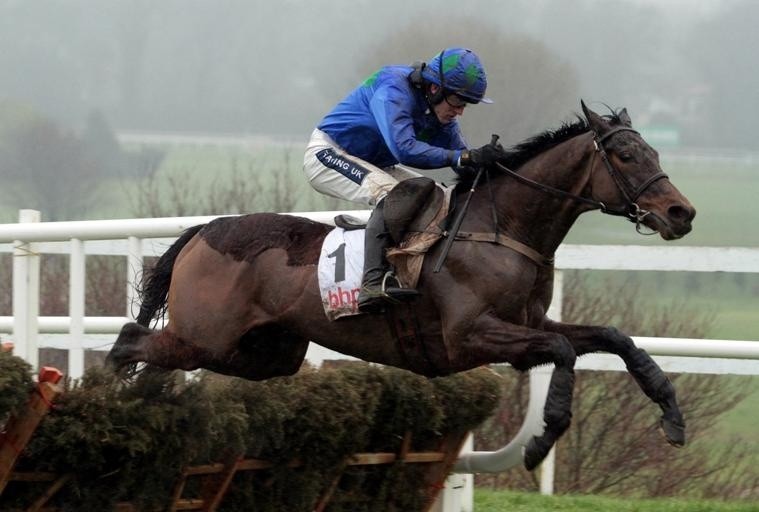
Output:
[420, 45, 488, 106]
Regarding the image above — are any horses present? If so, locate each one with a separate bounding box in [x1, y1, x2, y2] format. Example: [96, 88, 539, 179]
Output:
[103, 97, 697, 475]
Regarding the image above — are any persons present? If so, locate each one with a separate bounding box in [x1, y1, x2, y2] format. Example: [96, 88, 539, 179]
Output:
[302, 47, 501, 309]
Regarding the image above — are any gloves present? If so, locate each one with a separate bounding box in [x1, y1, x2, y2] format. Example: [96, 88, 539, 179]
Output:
[460, 144, 505, 167]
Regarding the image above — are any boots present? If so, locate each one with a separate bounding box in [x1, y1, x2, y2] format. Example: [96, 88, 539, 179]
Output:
[357, 193, 420, 311]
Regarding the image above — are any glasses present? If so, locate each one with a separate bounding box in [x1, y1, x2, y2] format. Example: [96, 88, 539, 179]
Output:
[445, 90, 468, 109]
